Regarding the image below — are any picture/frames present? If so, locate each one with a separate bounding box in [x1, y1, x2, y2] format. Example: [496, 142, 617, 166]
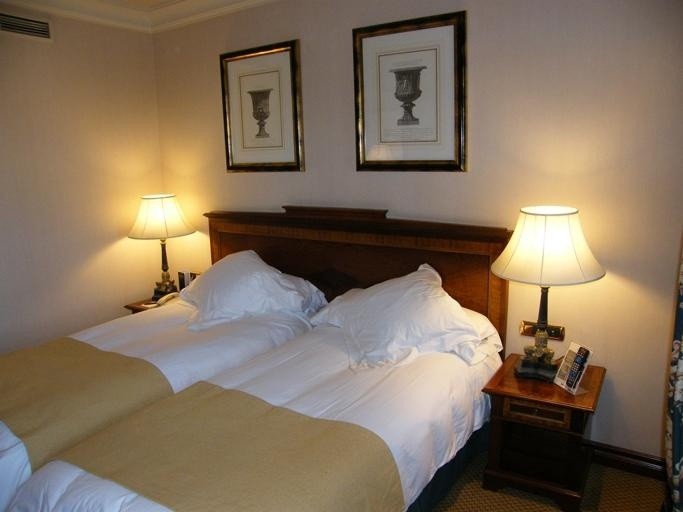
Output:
[220, 39, 305, 174]
[352, 11, 468, 171]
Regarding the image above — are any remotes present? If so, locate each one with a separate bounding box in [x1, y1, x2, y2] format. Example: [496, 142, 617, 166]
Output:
[566, 347, 589, 388]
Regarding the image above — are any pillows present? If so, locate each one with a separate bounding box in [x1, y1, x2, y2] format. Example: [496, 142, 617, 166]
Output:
[179, 250, 327, 331]
[310, 264, 501, 372]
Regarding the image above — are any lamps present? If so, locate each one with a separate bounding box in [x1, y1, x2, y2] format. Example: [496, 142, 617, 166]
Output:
[490, 205, 606, 383]
[128, 194, 196, 300]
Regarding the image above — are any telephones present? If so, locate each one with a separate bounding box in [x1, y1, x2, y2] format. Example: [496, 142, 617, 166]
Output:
[156, 292, 180, 306]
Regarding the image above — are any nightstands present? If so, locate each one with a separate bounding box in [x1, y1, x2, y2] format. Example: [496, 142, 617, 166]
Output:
[482, 354, 606, 512]
[125, 298, 155, 313]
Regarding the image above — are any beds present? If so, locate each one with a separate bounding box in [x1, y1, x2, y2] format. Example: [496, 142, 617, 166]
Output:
[0, 205, 513, 512]
